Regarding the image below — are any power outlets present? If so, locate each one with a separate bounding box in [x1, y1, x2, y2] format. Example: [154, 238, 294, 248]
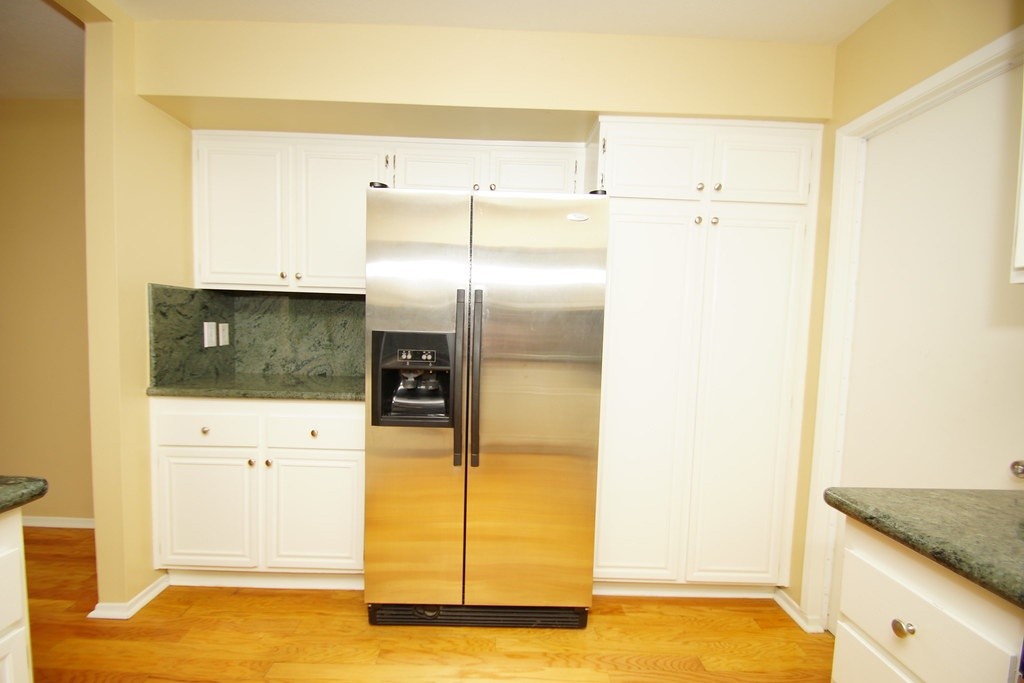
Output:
[218, 323, 230, 347]
[203, 322, 218, 348]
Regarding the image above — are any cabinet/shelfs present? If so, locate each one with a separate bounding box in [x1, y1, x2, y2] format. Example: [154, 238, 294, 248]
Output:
[148, 394, 365, 574]
[593, 114, 823, 589]
[192, 129, 386, 297]
[388, 136, 586, 194]
[830, 512, 1024, 682]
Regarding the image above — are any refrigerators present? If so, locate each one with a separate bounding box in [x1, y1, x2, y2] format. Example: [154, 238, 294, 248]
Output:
[357, 184, 611, 632]
